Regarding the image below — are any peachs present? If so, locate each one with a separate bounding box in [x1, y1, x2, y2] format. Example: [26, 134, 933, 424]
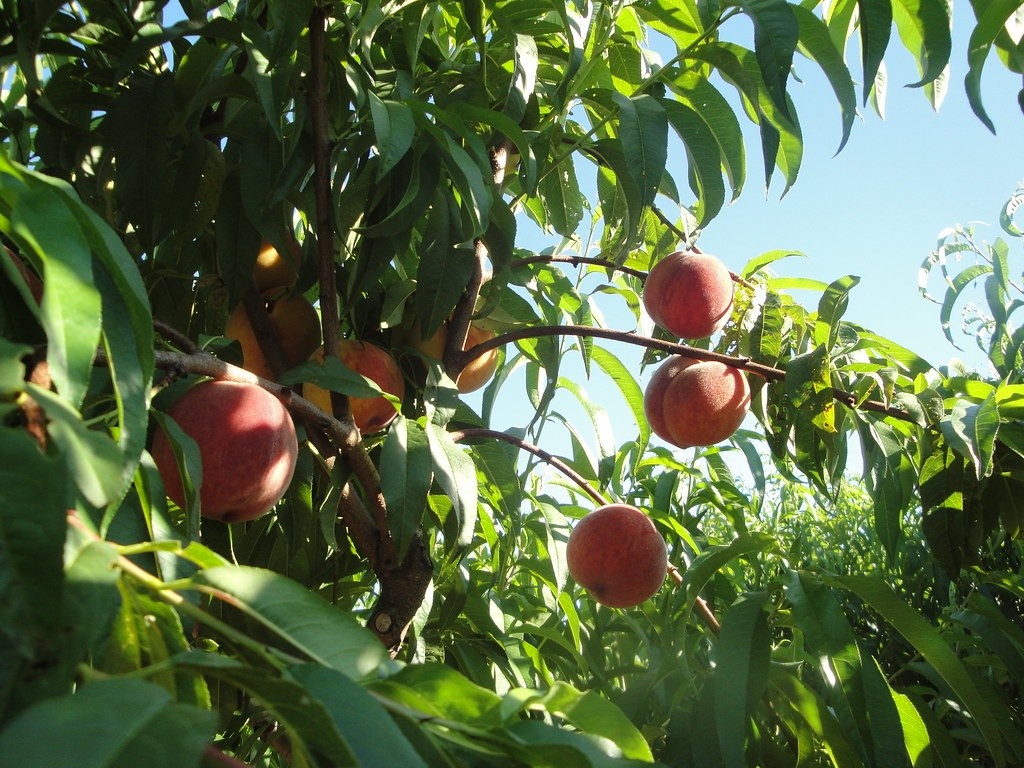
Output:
[0, 226, 750, 611]
